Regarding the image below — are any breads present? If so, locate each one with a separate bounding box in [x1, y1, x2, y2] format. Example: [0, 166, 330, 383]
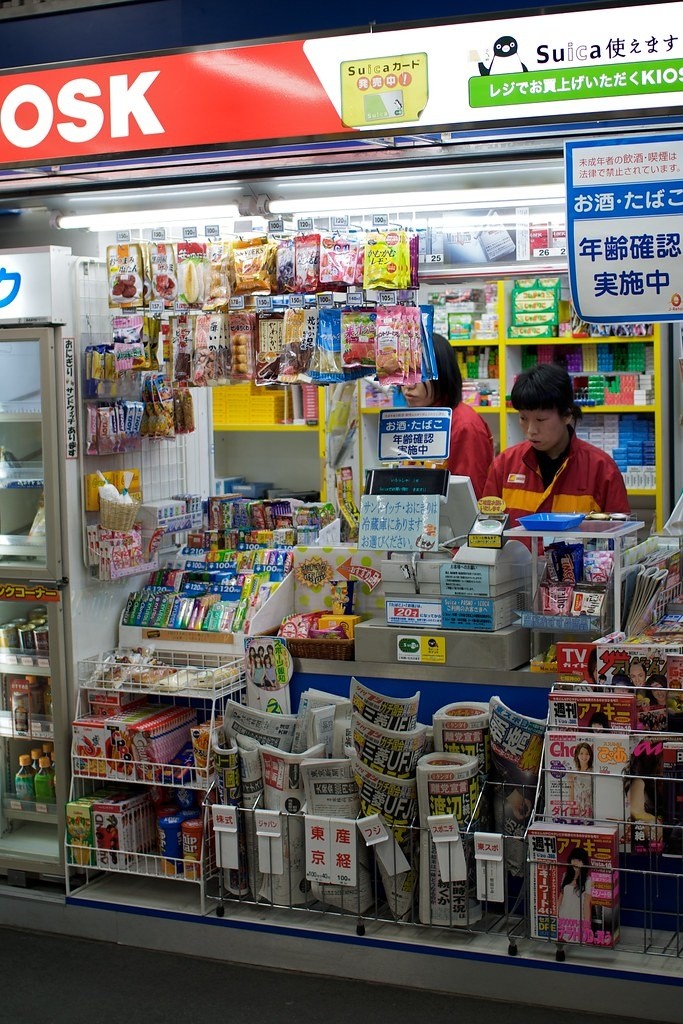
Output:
[100, 649, 241, 691]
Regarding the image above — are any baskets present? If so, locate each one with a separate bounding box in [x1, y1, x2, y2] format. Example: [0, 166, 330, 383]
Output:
[255, 625, 355, 660]
[99, 496, 141, 533]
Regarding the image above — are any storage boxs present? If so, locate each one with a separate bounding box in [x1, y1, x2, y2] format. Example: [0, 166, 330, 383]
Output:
[445, 288, 485, 313]
[214, 384, 319, 425]
[232, 483, 273, 497]
[573, 414, 655, 489]
[508, 275, 561, 338]
[215, 477, 244, 494]
[519, 346, 656, 407]
[64, 690, 197, 870]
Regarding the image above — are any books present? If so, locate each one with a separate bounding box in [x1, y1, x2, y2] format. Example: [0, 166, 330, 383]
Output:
[529, 548, 683, 948]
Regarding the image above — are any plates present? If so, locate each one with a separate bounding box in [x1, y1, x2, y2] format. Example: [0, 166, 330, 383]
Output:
[515, 513, 585, 531]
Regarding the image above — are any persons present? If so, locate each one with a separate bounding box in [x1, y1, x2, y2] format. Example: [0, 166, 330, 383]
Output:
[483, 363, 629, 555]
[248, 645, 278, 688]
[397, 332, 493, 501]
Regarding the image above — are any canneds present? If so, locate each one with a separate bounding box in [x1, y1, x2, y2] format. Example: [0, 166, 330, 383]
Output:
[0, 607, 49, 657]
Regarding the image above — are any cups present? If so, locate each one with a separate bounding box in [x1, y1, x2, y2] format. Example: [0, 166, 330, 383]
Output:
[157, 806, 203, 881]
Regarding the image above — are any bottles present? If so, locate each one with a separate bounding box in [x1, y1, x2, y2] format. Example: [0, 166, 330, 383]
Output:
[29, 492, 46, 537]
[15, 744, 54, 804]
[22, 676, 53, 734]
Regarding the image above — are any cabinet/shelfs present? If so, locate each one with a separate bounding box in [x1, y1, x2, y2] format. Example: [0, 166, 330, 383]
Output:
[360, 265, 669, 532]
[528, 683, 683, 961]
[64, 646, 246, 912]
[118, 518, 341, 654]
[212, 381, 326, 511]
